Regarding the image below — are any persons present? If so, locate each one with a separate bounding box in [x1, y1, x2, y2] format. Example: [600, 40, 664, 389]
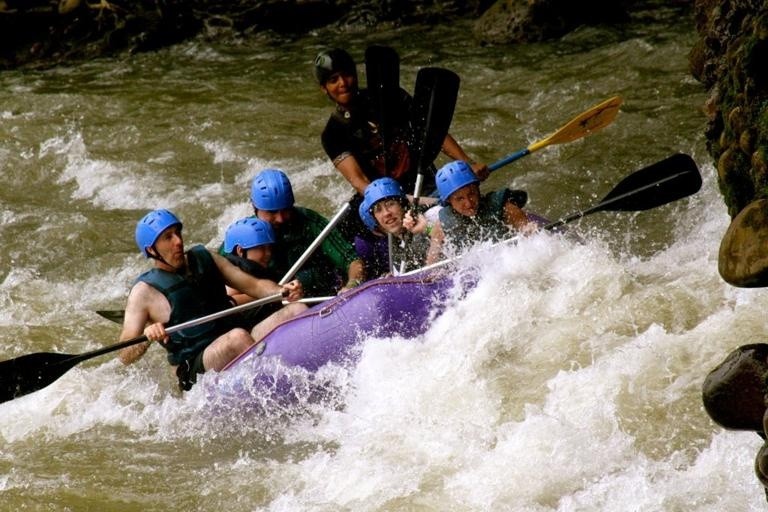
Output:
[427, 160, 532, 266]
[314, 46, 488, 200]
[215, 216, 277, 313]
[117, 208, 309, 394]
[218, 168, 365, 298]
[359, 200, 390, 276]
[364, 177, 445, 275]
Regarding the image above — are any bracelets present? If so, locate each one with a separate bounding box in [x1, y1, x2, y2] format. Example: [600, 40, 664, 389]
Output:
[344, 279, 363, 290]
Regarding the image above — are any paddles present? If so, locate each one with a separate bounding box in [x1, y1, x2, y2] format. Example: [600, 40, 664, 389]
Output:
[95, 296, 339, 327]
[1, 283, 295, 404]
[485, 95, 621, 172]
[401, 153, 703, 277]
[399, 68, 460, 274]
[362, 45, 400, 275]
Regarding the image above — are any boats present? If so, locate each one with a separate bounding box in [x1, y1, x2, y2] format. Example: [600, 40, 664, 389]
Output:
[193, 274, 487, 413]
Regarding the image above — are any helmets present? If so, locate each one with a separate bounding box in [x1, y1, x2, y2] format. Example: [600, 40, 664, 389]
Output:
[357, 179, 402, 231]
[225, 218, 273, 255]
[252, 170, 294, 212]
[134, 209, 183, 258]
[436, 160, 479, 206]
[314, 46, 356, 84]
